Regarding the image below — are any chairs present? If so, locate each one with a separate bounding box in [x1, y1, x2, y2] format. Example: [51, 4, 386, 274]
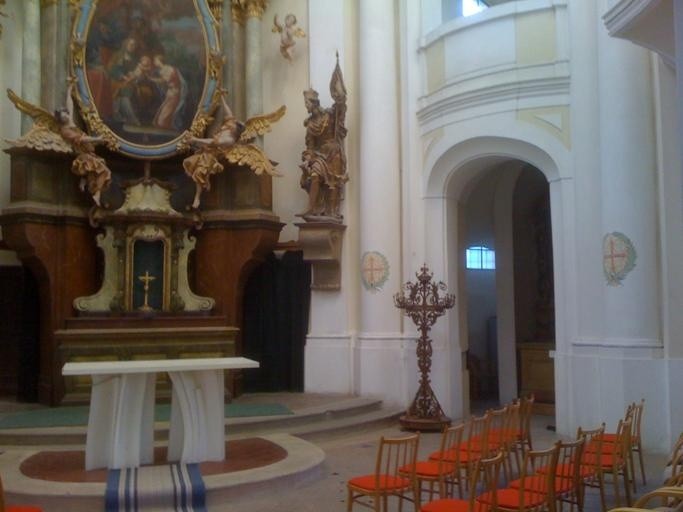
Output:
[348, 430, 420, 511]
[398, 392, 683, 512]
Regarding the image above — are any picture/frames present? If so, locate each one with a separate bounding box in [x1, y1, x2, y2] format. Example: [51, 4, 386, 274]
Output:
[69, 0, 223, 161]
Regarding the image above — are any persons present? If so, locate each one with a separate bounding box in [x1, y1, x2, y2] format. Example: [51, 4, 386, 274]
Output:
[182, 87, 247, 208]
[110, 36, 143, 125]
[124, 57, 150, 82]
[294, 87, 346, 222]
[146, 52, 187, 129]
[279, 14, 300, 52]
[54, 77, 114, 208]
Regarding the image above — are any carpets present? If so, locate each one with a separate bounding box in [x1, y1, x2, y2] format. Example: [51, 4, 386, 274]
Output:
[0, 402, 295, 430]
